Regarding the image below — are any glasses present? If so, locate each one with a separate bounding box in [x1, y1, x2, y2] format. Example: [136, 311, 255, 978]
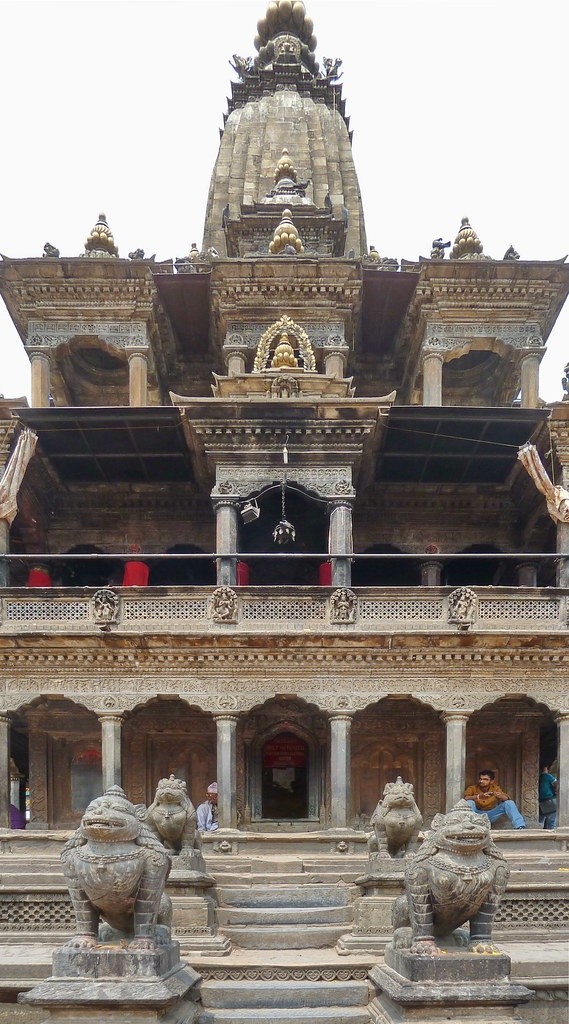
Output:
[548, 768, 551, 771]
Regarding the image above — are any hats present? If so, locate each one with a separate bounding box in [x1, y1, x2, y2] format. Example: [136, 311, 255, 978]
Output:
[207, 782, 217, 793]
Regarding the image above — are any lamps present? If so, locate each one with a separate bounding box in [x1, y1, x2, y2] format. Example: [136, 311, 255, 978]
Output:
[241, 498, 260, 523]
[273, 473, 296, 544]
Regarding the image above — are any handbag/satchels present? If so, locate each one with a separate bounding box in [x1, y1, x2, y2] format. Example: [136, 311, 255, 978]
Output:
[539, 798, 557, 814]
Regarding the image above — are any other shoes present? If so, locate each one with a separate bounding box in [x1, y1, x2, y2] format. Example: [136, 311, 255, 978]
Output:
[514, 827, 525, 829]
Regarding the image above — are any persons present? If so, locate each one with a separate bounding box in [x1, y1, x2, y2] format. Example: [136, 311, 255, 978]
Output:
[10, 804, 27, 830]
[196, 782, 219, 834]
[463, 770, 526, 831]
[539, 761, 557, 830]
